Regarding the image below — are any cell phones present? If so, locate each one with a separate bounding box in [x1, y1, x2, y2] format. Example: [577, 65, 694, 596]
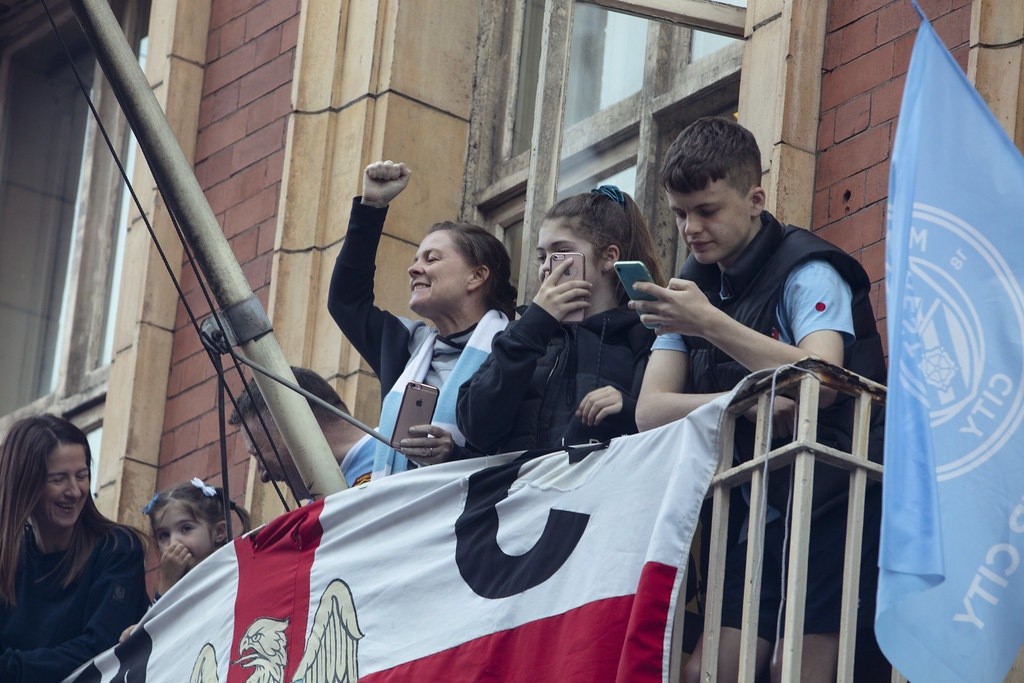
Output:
[613, 261, 658, 329]
[389, 381, 439, 449]
[549, 252, 586, 323]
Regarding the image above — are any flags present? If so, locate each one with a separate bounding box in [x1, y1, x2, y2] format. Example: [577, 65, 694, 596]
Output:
[873, 17, 1024, 683]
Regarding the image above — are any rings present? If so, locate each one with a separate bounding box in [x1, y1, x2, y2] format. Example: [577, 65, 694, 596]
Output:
[429, 448, 432, 457]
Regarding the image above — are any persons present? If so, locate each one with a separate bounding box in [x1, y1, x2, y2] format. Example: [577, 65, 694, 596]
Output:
[628, 115, 888, 683]
[327, 159, 518, 482]
[228, 366, 379, 505]
[118, 477, 251, 642]
[456, 185, 669, 457]
[0, 413, 152, 683]
